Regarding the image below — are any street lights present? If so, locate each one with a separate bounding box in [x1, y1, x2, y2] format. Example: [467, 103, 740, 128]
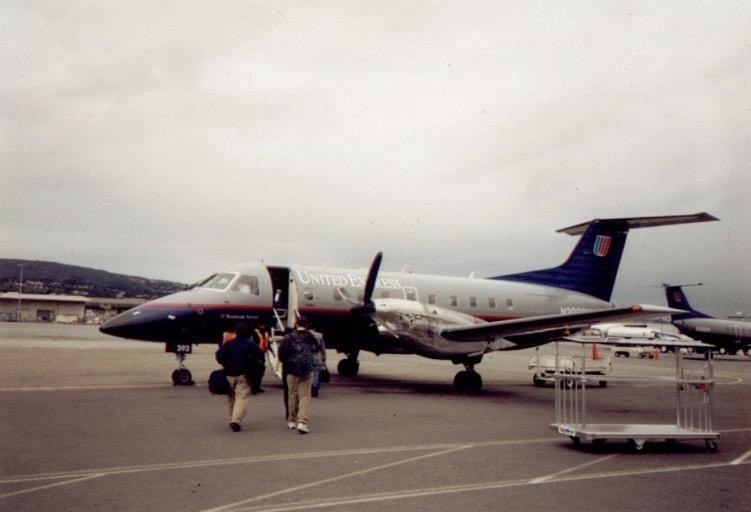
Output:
[16, 262, 26, 322]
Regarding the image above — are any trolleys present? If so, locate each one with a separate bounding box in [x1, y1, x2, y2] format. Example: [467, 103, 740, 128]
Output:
[527, 341, 612, 387]
[545, 331, 720, 454]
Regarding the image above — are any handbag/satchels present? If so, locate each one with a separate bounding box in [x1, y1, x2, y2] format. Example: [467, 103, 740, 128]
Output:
[208, 369, 232, 395]
[319, 365, 330, 383]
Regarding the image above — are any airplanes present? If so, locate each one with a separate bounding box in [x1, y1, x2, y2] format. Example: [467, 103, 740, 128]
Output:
[564, 282, 751, 361]
[95, 209, 724, 395]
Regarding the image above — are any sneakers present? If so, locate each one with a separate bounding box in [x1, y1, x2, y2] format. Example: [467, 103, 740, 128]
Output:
[297, 423, 309, 433]
[288, 421, 296, 429]
[229, 422, 240, 432]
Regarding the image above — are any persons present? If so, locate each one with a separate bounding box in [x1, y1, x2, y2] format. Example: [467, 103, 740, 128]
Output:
[218, 325, 237, 347]
[280, 317, 322, 433]
[251, 319, 278, 395]
[216, 324, 266, 432]
[283, 326, 294, 420]
[305, 322, 330, 398]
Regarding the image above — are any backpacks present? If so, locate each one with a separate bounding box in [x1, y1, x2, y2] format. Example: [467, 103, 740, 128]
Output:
[287, 332, 315, 377]
